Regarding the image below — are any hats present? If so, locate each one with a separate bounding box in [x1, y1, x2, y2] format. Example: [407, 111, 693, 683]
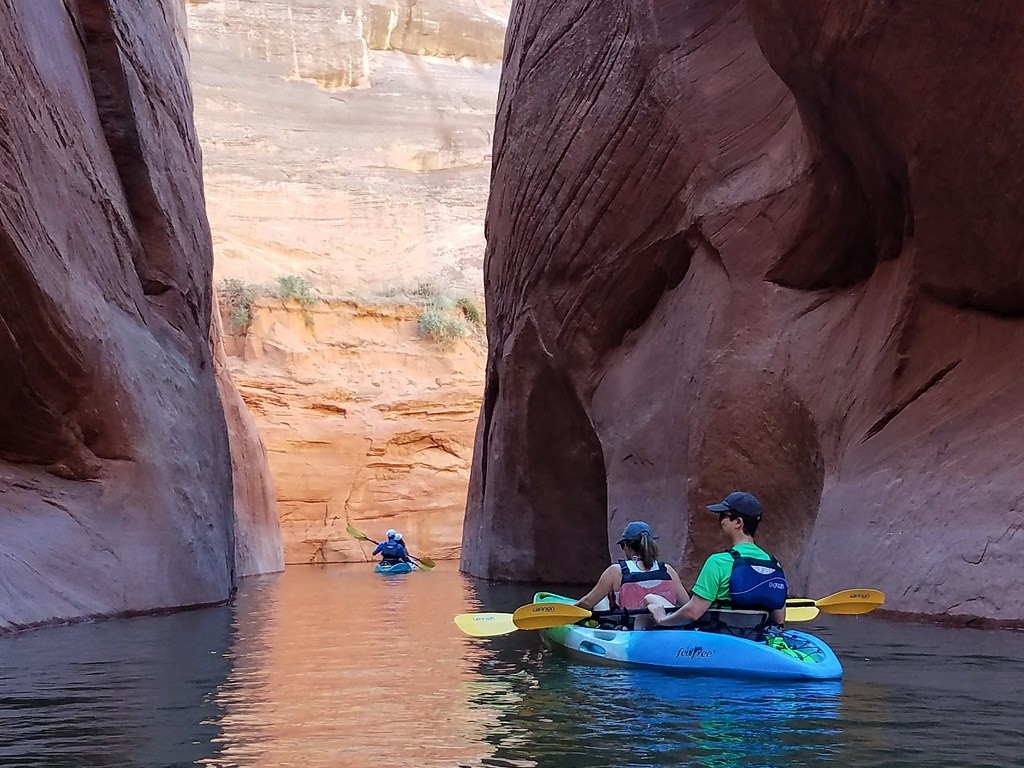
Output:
[386, 529, 396, 537]
[616, 521, 652, 545]
[706, 492, 763, 520]
[394, 534, 402, 540]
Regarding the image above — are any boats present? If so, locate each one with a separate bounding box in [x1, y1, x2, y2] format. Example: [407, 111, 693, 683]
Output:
[533, 592, 844, 679]
[373, 555, 417, 575]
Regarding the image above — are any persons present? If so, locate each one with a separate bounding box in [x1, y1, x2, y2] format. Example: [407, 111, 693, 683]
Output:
[373, 529, 410, 566]
[647, 493, 787, 630]
[573, 520, 690, 611]
[394, 534, 409, 556]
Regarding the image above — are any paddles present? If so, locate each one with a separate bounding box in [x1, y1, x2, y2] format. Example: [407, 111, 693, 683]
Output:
[513, 588, 887, 630]
[452, 598, 821, 637]
[345, 526, 436, 572]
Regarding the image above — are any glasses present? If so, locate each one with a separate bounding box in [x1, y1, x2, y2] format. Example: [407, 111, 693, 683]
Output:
[719, 513, 734, 520]
[620, 542, 630, 550]
